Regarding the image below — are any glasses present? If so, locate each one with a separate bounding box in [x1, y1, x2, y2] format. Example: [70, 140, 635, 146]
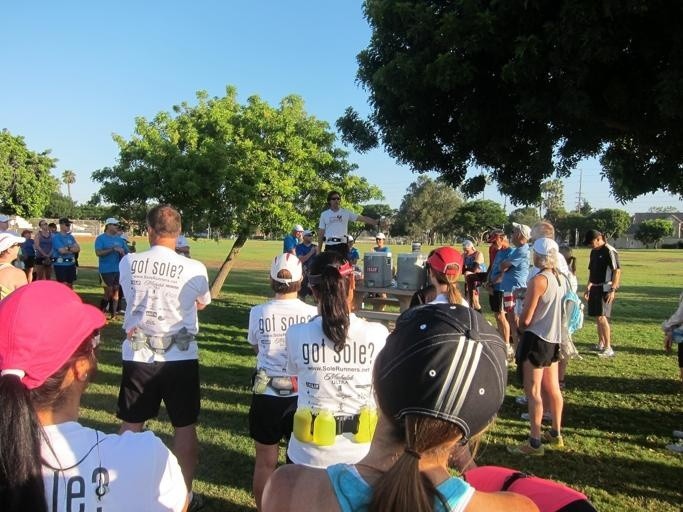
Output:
[331, 197, 340, 200]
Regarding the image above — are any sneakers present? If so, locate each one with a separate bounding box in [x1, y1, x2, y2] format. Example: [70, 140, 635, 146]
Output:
[665, 445, 683, 452]
[507, 381, 566, 456]
[591, 344, 605, 350]
[598, 346, 613, 357]
[673, 431, 683, 438]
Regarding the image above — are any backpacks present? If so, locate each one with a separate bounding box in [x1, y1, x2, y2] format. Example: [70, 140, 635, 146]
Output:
[561, 289, 584, 360]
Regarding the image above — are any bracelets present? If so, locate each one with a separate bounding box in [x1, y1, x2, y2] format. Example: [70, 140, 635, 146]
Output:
[584, 288, 590, 294]
[608, 287, 617, 292]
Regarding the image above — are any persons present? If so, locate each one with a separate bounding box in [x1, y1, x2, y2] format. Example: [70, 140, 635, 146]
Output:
[582, 229, 620, 358]
[110, 205, 210, 511]
[661, 296, 682, 453]
[519, 237, 566, 456]
[411, 221, 578, 389]
[317, 191, 379, 261]
[0, 215, 136, 322]
[247, 253, 321, 511]
[175, 234, 190, 258]
[284, 225, 395, 312]
[259, 304, 542, 511]
[0, 280, 190, 511]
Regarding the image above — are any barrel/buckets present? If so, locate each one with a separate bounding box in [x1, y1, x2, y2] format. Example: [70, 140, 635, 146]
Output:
[397, 254, 429, 291]
[364, 252, 393, 289]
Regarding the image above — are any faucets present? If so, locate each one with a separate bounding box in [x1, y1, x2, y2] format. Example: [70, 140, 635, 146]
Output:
[366, 280, 374, 290]
[401, 283, 407, 290]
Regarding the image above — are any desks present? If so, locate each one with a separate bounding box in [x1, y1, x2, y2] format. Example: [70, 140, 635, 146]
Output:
[356, 285, 415, 311]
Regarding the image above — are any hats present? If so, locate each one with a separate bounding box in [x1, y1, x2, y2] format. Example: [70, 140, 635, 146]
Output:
[463, 222, 600, 256]
[0, 280, 106, 390]
[292, 225, 385, 242]
[428, 247, 463, 275]
[0, 215, 120, 254]
[374, 303, 507, 446]
[270, 253, 302, 283]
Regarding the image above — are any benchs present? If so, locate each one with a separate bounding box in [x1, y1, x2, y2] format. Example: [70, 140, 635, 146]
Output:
[353, 308, 403, 322]
